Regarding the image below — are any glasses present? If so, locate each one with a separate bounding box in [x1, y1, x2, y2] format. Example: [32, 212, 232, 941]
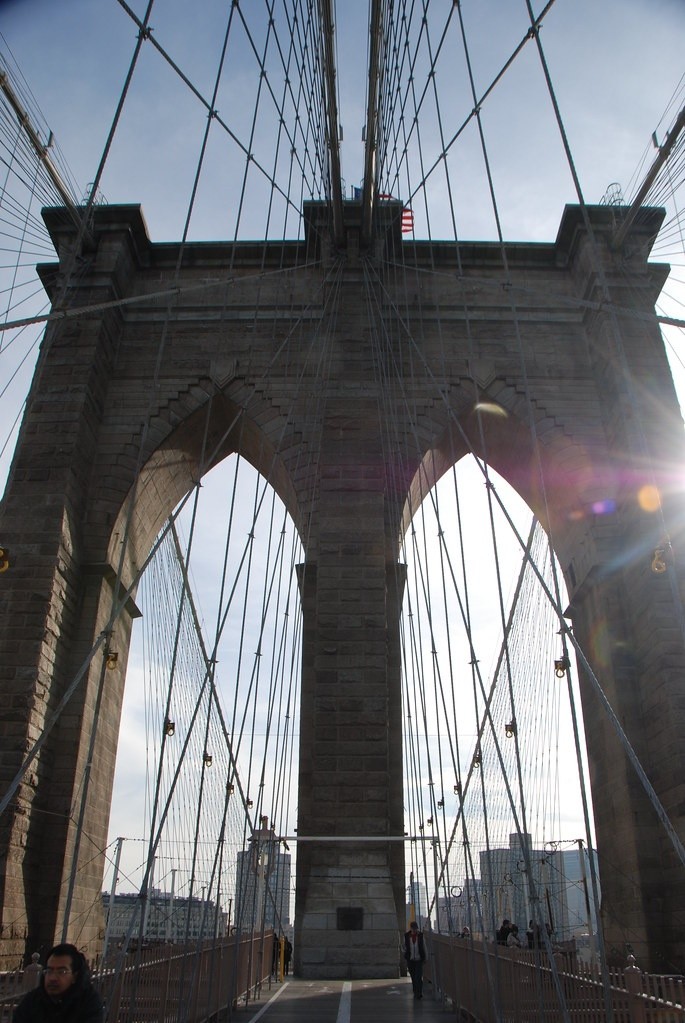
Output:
[42, 968, 73, 976]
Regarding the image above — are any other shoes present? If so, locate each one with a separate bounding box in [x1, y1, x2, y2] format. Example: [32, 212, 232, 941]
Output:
[414, 994, 422, 1000]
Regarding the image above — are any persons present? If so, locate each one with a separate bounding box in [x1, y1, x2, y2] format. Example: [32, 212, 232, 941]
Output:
[461, 927, 470, 938]
[272, 933, 293, 976]
[403, 920, 429, 1000]
[498, 920, 552, 949]
[14, 943, 104, 1023]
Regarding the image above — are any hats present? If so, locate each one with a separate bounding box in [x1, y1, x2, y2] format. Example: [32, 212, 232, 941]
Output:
[410, 921, 419, 929]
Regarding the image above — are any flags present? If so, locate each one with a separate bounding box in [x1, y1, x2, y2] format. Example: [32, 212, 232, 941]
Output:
[355, 188, 414, 233]
